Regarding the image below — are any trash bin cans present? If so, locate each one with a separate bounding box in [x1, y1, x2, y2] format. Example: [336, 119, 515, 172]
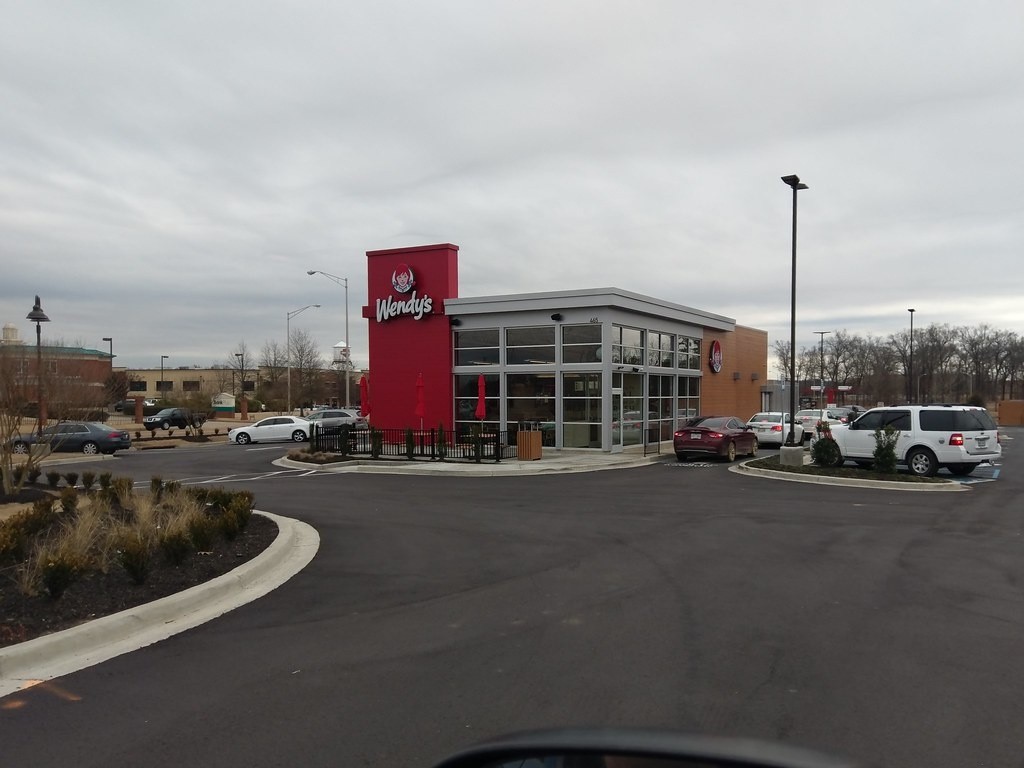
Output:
[517, 418, 543, 461]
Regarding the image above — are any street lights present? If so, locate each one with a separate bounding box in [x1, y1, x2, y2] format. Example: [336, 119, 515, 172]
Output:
[27, 294, 51, 434]
[285, 304, 321, 414]
[101, 337, 113, 374]
[306, 270, 351, 411]
[235, 353, 248, 421]
[160, 355, 170, 397]
[782, 175, 809, 447]
[908, 308, 915, 404]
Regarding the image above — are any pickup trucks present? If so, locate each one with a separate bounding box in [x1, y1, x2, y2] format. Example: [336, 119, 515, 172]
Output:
[143, 407, 208, 432]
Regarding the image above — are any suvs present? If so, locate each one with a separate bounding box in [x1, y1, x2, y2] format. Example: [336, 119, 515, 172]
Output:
[823, 402, 1001, 478]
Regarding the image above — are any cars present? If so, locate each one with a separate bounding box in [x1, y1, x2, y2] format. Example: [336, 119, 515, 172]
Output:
[673, 414, 758, 463]
[3, 419, 131, 456]
[794, 409, 843, 438]
[746, 411, 806, 447]
[612, 408, 662, 444]
[227, 416, 326, 444]
[827, 408, 853, 424]
[305, 408, 370, 431]
[115, 398, 137, 410]
[843, 405, 864, 417]
